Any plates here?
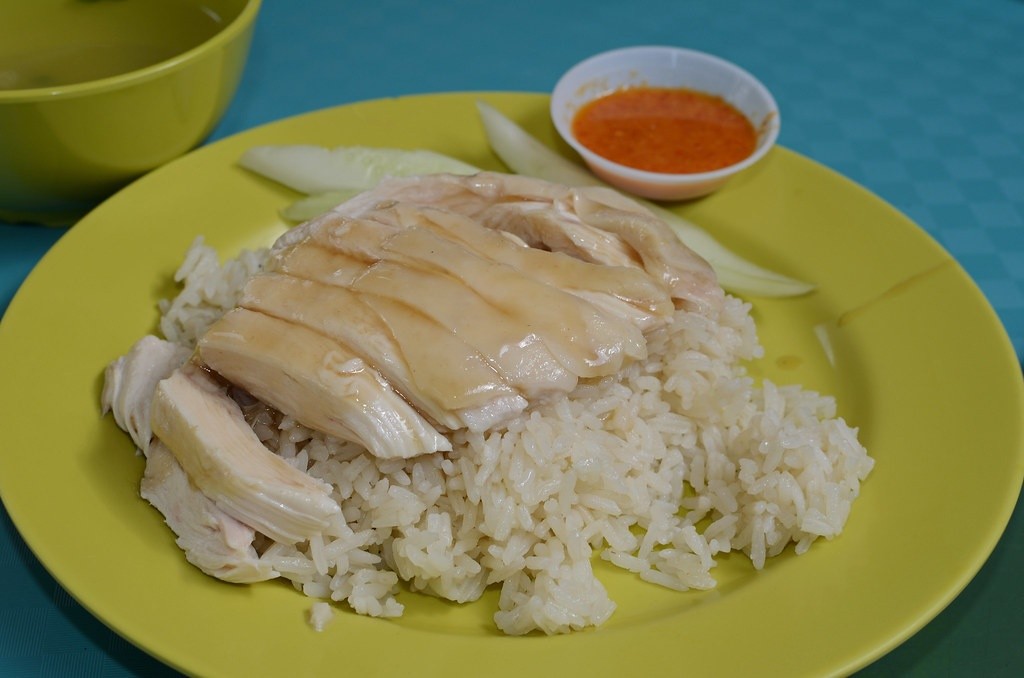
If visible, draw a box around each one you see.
[0,90,1024,678]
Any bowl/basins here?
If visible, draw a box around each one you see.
[0,0,261,227]
[549,45,781,200]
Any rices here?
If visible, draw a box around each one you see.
[155,218,878,637]
